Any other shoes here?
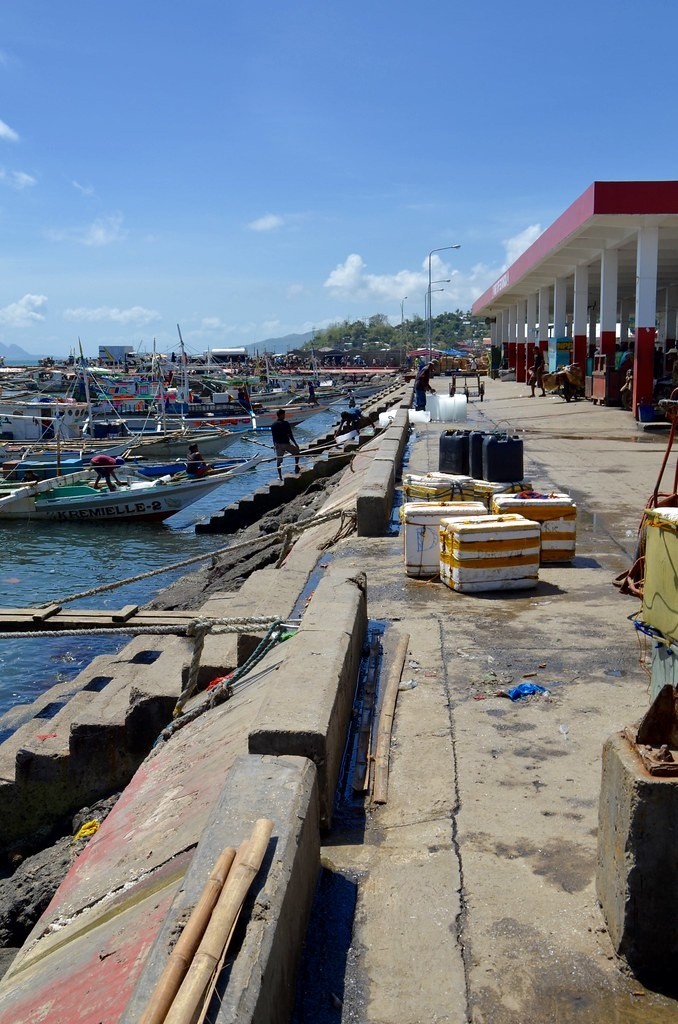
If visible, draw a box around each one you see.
[529,395,535,397]
[539,394,546,397]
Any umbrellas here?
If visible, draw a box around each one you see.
[440,349,467,357]
[318,347,340,355]
[406,349,442,358]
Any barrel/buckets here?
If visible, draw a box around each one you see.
[437,428,524,482]
[637,402,654,422]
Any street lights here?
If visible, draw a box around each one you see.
[427,244,462,380]
[423,288,444,364]
[399,296,409,367]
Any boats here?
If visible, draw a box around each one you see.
[0,321,395,523]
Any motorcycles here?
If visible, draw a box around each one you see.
[554,363,581,402]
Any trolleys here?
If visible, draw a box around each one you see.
[450,372,485,404]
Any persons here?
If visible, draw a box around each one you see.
[20,470,41,482]
[334,408,375,439]
[273,356,377,369]
[526,346,545,397]
[270,409,299,481]
[470,358,477,371]
[307,381,318,407]
[90,455,124,492]
[38,357,266,376]
[185,443,210,479]
[414,359,439,412]
[238,388,246,404]
[613,342,664,412]
[345,389,355,408]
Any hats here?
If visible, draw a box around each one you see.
[116,458,125,465]
[428,359,440,367]
